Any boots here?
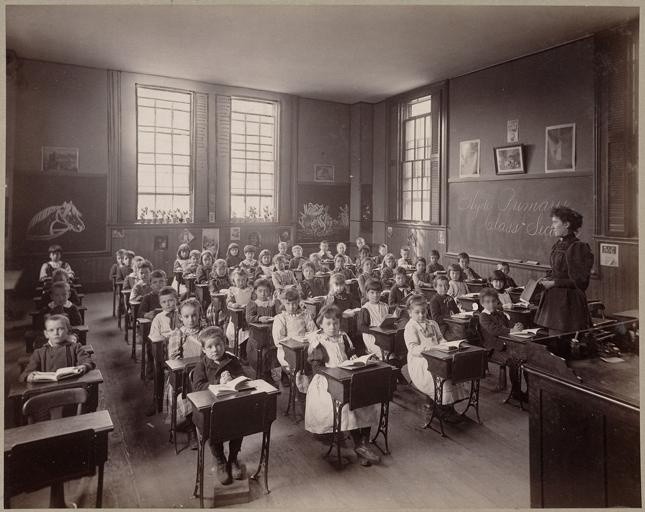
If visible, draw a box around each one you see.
[228,448,244,478]
[216,452,231,485]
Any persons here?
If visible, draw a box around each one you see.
[533,206,595,337]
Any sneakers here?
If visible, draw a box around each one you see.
[512,391,528,402]
[281,371,291,388]
[266,377,281,394]
[189,431,198,450]
[143,402,162,417]
[354,435,381,466]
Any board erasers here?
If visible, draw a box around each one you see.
[527,261,540,265]
[513,259,523,263]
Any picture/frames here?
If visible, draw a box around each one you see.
[492,143,527,176]
[312,163,336,184]
[39,145,80,174]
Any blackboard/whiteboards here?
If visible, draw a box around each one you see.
[10,171,112,256]
[443,168,601,280]
[296,183,373,246]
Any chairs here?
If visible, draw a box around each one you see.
[370,246,639,436]
[4,246,400,510]
